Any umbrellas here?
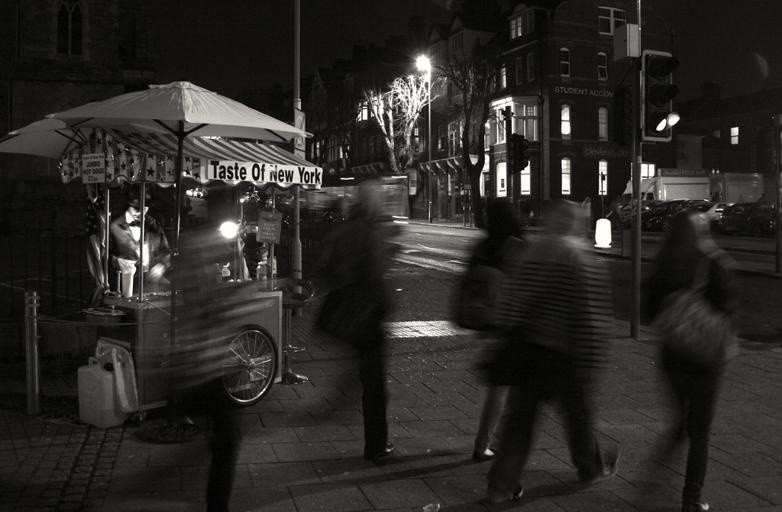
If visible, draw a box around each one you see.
[44,81,313,420]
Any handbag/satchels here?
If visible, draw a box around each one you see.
[655,290,731,373]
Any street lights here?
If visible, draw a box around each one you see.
[414,52,432,221]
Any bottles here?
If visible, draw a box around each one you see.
[221,264,230,284]
[257,261,266,281]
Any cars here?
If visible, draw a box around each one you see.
[594,197,781,232]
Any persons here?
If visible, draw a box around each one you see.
[646,203,735,512]
[312,179,398,465]
[110,193,172,297]
[468,194,620,505]
[176,186,253,511]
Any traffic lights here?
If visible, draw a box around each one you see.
[641,49,683,142]
[508,132,530,173]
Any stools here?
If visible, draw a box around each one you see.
[260,276,315,387]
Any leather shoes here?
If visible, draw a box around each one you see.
[681,502,709,512]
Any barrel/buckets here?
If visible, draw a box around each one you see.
[76,356,130,430]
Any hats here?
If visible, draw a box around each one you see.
[129,194,149,207]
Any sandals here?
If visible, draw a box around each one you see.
[473,448,498,461]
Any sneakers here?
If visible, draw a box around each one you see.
[489,485,524,504]
[364,441,396,460]
[577,446,623,488]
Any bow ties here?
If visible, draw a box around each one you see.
[130,220,141,226]
